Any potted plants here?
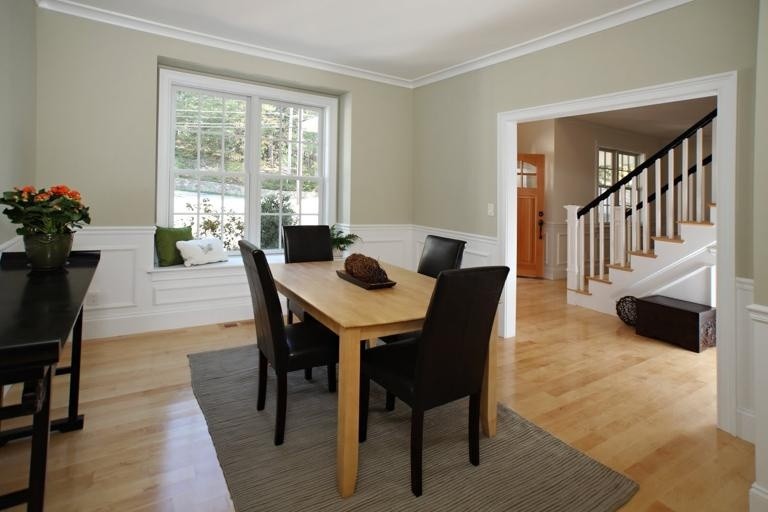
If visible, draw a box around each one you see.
[327,224,357,256]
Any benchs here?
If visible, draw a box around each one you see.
[633,293,715,354]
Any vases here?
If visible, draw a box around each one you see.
[22,230,73,270]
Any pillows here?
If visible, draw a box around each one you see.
[176,236,229,269]
[154,224,193,267]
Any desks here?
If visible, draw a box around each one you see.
[266,257,503,500]
[0,248,103,512]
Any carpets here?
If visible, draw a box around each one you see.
[180,342,639,512]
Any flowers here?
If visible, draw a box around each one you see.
[0,186,92,234]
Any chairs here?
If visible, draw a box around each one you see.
[373,233,468,417]
[233,237,366,444]
[279,224,334,382]
[359,264,510,496]
